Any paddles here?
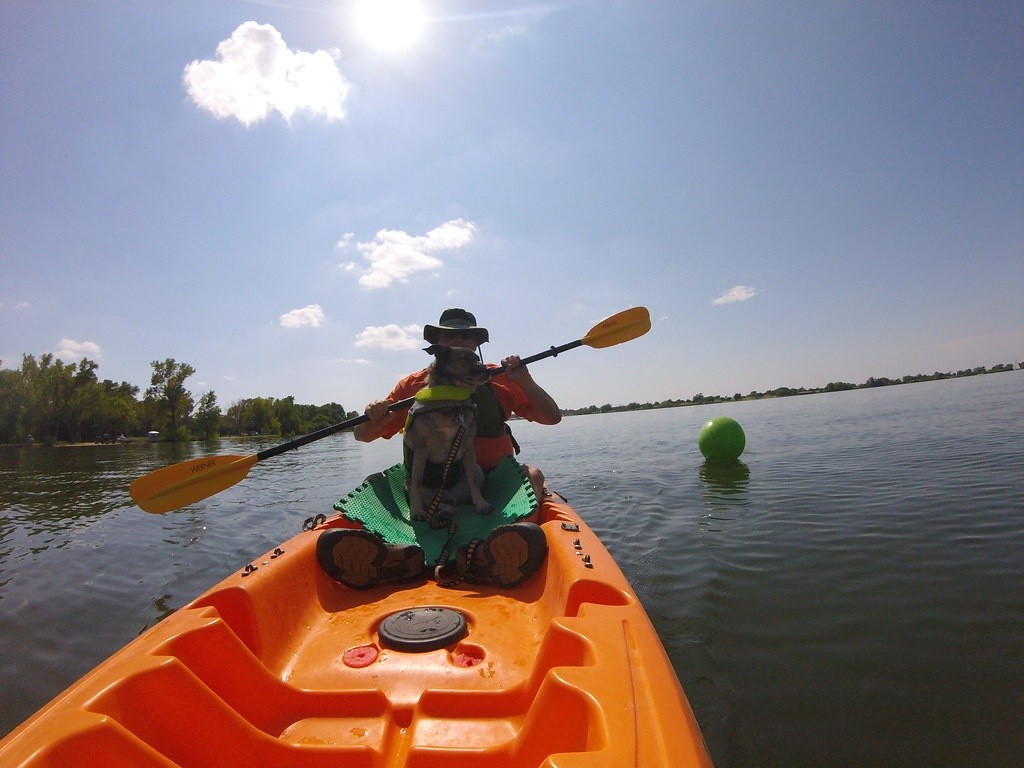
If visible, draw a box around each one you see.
[130,304,652,516]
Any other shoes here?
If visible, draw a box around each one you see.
[449,521,547,589]
[316,528,424,590]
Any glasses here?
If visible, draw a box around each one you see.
[438,331,474,341]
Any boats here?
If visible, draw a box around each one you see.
[0,461,716,767]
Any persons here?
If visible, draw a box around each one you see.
[316,308,562,589]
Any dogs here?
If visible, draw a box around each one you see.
[403,344,495,520]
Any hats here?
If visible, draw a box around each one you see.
[423,309,489,345]
